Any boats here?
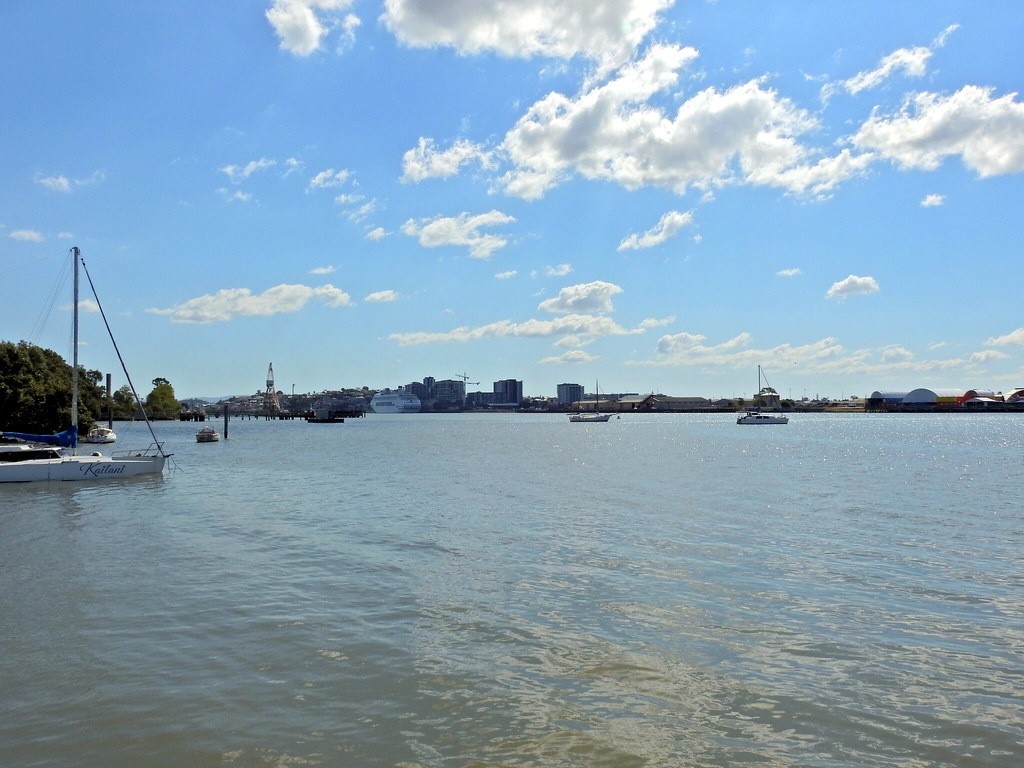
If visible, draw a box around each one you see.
[84,427,117,443]
[195,426,222,442]
[368,386,423,414]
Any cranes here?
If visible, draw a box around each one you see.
[455,372,480,386]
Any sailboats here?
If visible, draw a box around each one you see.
[0,243,176,484]
[569,380,613,422]
[736,365,790,424]
